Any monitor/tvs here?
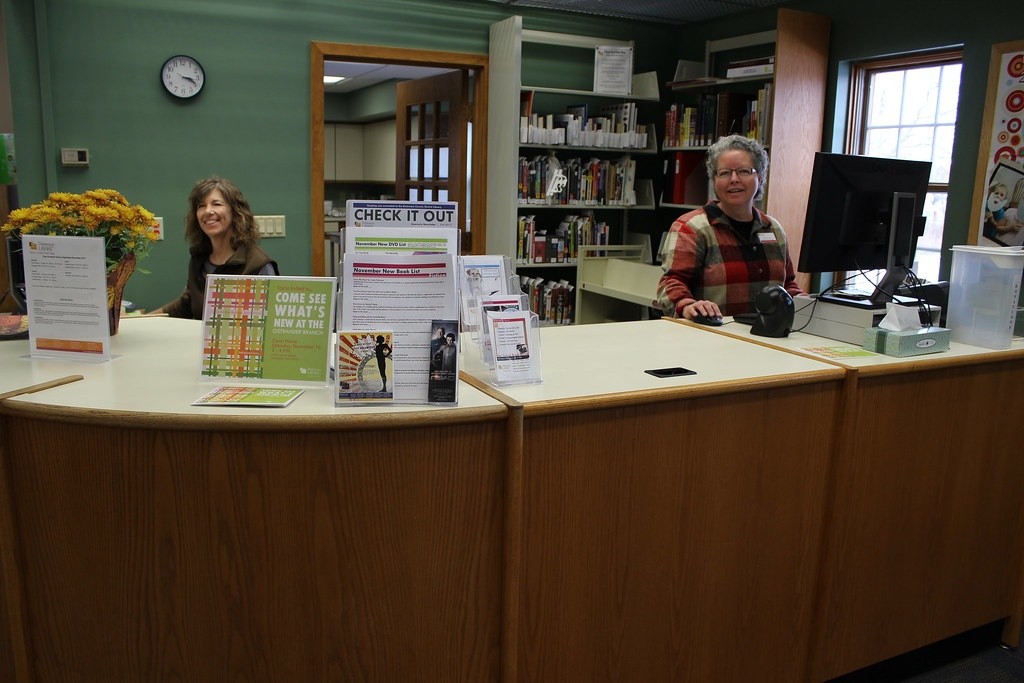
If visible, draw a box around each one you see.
[796,152,933,309]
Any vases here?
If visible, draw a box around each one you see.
[106,251,137,336]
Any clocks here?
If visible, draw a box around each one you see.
[159,55,207,102]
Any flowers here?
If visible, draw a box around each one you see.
[0,187,160,278]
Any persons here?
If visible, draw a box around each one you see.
[120,179,279,320]
[657,135,808,320]
[431,328,457,377]
[983,183,1024,247]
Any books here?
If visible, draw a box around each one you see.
[727,56,775,78]
[518,156,636,206]
[754,172,767,212]
[520,90,647,148]
[518,211,610,263]
[520,277,574,325]
[665,77,724,86]
[708,177,718,201]
[664,83,772,146]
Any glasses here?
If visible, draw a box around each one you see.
[714,168,757,180]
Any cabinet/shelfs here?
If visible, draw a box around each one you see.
[655,8,832,294]
[486,16,661,327]
[323,118,397,184]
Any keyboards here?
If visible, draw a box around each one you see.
[733,312,759,325]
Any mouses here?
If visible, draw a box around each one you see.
[692,309,722,327]
